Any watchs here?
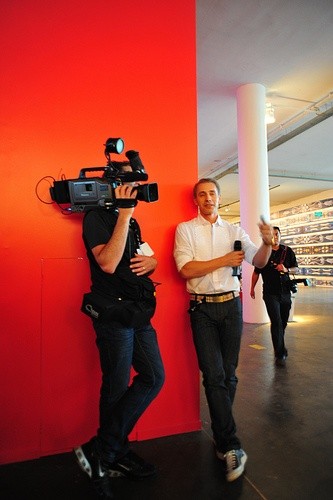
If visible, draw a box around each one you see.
[286,267,291,274]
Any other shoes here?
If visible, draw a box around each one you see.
[277,356,284,366]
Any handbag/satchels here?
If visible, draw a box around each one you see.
[82,292,153,329]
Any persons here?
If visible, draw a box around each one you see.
[249,226,300,367]
[69,159,165,481]
[172,179,274,483]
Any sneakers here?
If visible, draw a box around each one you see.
[74,436,109,480]
[102,451,155,479]
[214,438,225,461]
[224,448,248,481]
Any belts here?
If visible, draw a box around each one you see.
[190,291,239,303]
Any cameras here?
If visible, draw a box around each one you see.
[288,278,309,293]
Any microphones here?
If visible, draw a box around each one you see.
[231,240,242,275]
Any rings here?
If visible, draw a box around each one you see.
[142,266,146,270]
